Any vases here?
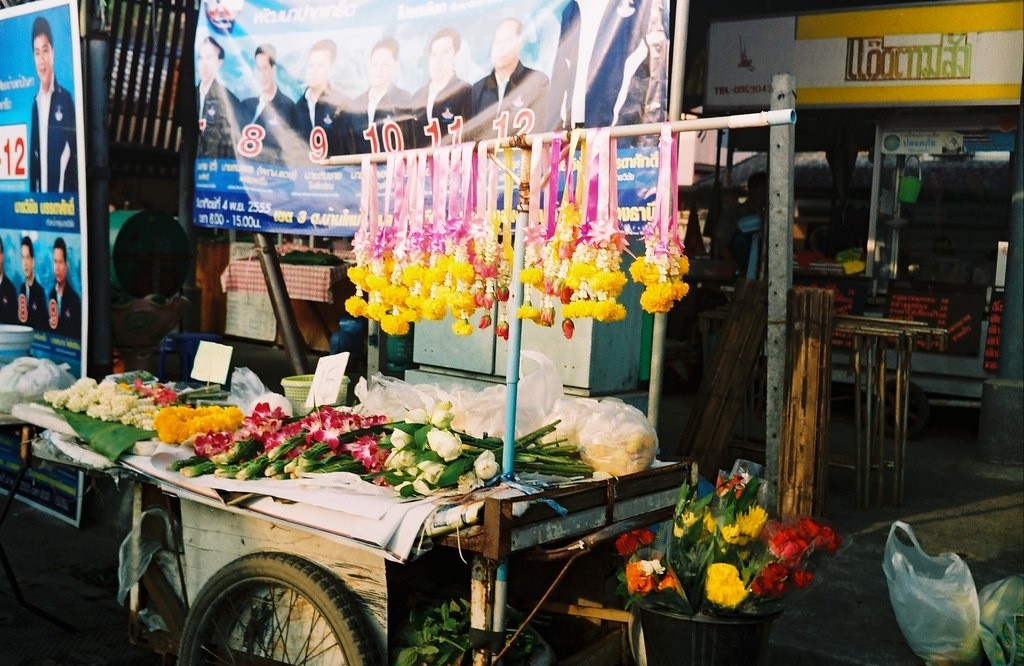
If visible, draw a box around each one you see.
[630,600,784,666]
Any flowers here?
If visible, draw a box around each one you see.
[614,463,841,619]
[345,203,689,340]
[44,375,594,502]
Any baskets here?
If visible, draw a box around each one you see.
[280,374,351,416]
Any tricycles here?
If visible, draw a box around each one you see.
[125,458,700,666]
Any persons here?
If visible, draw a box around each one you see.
[195,1,633,166]
[731,171,768,261]
[0,237,81,341]
[30,17,78,193]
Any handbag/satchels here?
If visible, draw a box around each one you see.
[354,349,659,476]
[882,520,1024,666]
